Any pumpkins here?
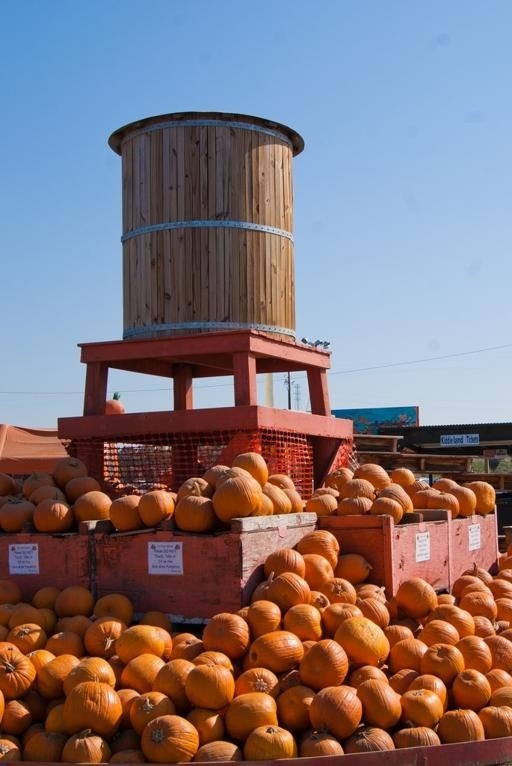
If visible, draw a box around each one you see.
[0,451,512,766]
[104,391,125,414]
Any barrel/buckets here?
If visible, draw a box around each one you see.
[105,111,306,340]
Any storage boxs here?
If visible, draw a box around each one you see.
[317,505,450,606]
[88,510,318,621]
[3,530,90,599]
[450,505,497,594]
